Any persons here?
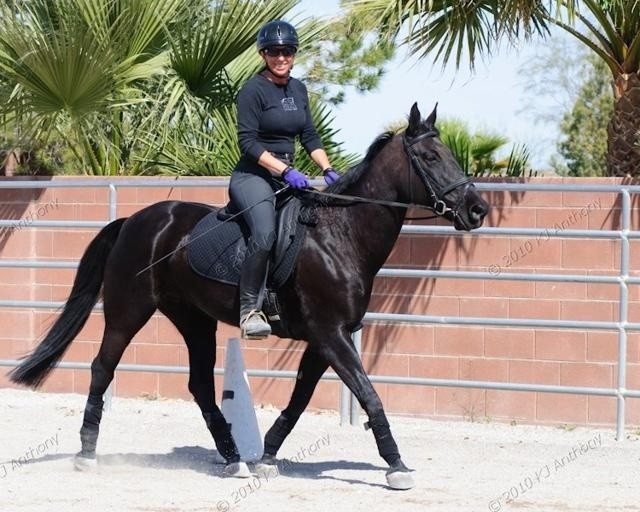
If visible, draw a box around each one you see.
[228,20,339,335]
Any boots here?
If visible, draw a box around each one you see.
[239,245,272,334]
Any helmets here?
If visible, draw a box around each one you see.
[257,21,298,53]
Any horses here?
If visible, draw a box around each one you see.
[6,102,488,491]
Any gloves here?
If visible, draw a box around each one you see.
[282,167,310,188]
[324,171,339,185]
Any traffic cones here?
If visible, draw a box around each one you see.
[215,339,264,465]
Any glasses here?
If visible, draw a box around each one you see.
[265,47,295,56]
